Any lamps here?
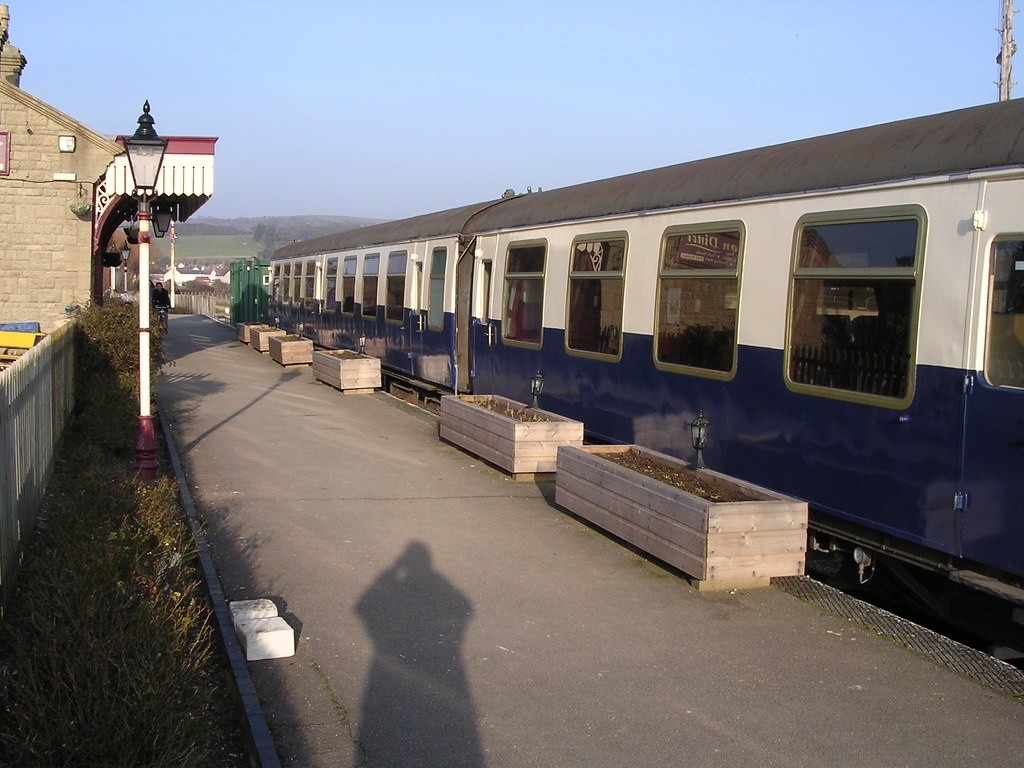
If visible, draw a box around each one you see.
[357,330,368,355]
[297,320,305,339]
[273,313,280,330]
[684,409,713,470]
[155,202,173,232]
[523,370,545,410]
[151,218,164,238]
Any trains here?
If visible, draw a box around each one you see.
[267,95,1023,610]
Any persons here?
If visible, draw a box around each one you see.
[149,279,170,335]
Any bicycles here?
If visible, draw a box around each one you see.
[154,302,170,333]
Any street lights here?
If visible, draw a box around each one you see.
[119,102,165,482]
